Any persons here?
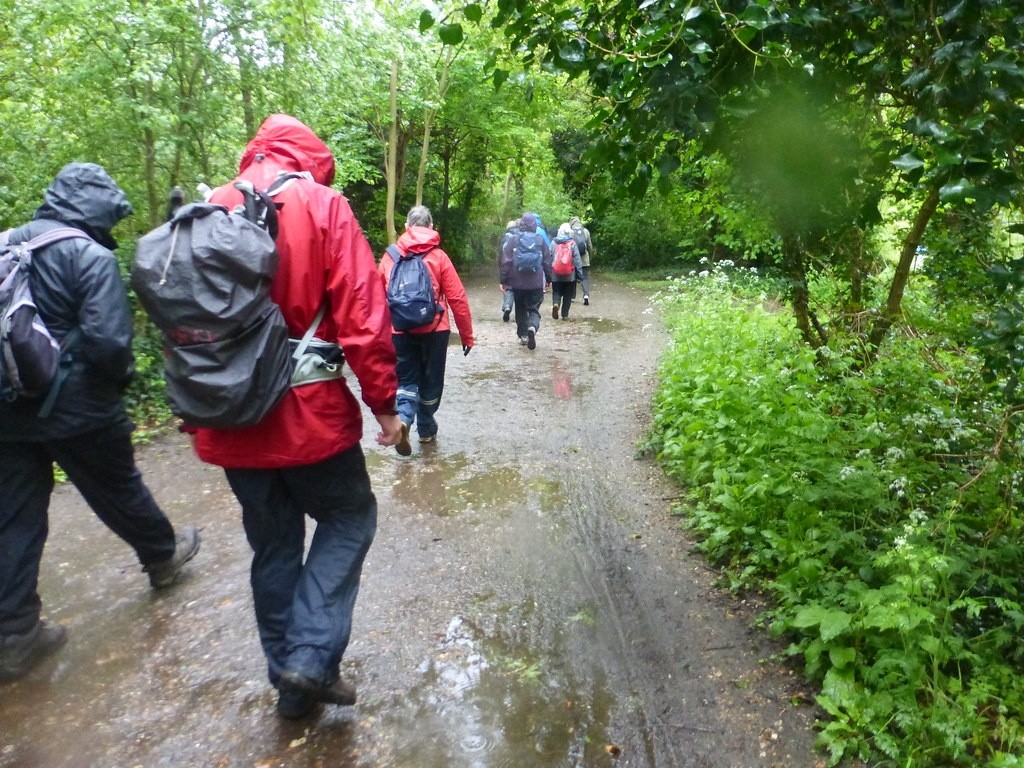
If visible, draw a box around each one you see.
[499,212,593,350]
[379,206,475,456]
[179,113,401,724]
[0,162,202,682]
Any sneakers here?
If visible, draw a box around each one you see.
[1,620,67,682]
[140,523,201,589]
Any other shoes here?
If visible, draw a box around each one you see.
[418,433,437,443]
[395,422,412,456]
[276,696,318,719]
[562,316,568,320]
[527,326,536,351]
[552,303,559,319]
[502,308,510,322]
[583,295,590,306]
[571,299,574,302]
[517,336,528,345]
[281,666,356,704]
[543,289,546,293]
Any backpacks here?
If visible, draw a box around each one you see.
[1,226,97,419]
[573,224,586,255]
[130,171,328,429]
[385,244,444,342]
[515,232,541,274]
[552,239,575,276]
